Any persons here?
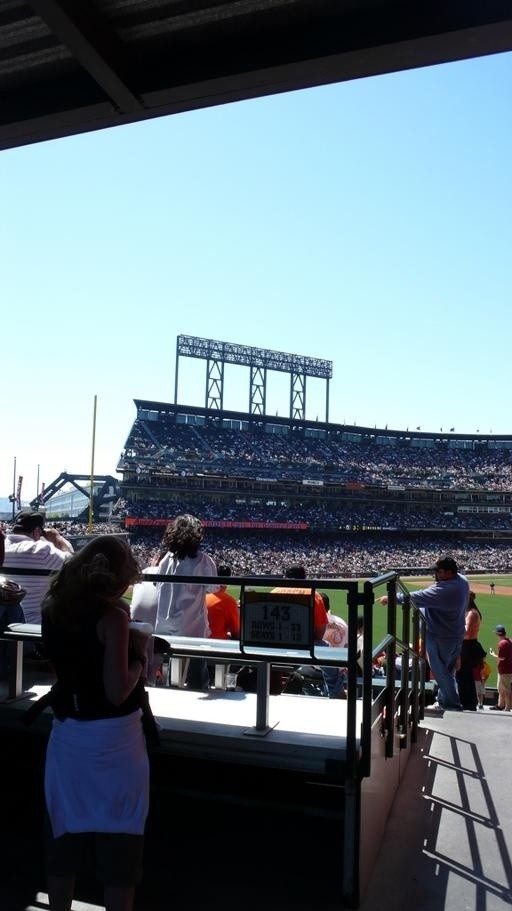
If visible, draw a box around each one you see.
[481,660,493,699]
[1,416,512,579]
[0,513,76,651]
[315,591,438,699]
[269,562,348,700]
[489,579,496,597]
[373,555,472,713]
[34,534,160,911]
[455,589,484,713]
[152,512,219,690]
[487,623,512,711]
[473,657,486,709]
[0,531,40,684]
[203,562,242,642]
[128,548,170,639]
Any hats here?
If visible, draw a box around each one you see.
[15,511,46,530]
[492,625,506,634]
[428,556,457,570]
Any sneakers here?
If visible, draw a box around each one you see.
[478,704,511,711]
[426,702,462,711]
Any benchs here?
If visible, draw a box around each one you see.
[4,622,434,911]
